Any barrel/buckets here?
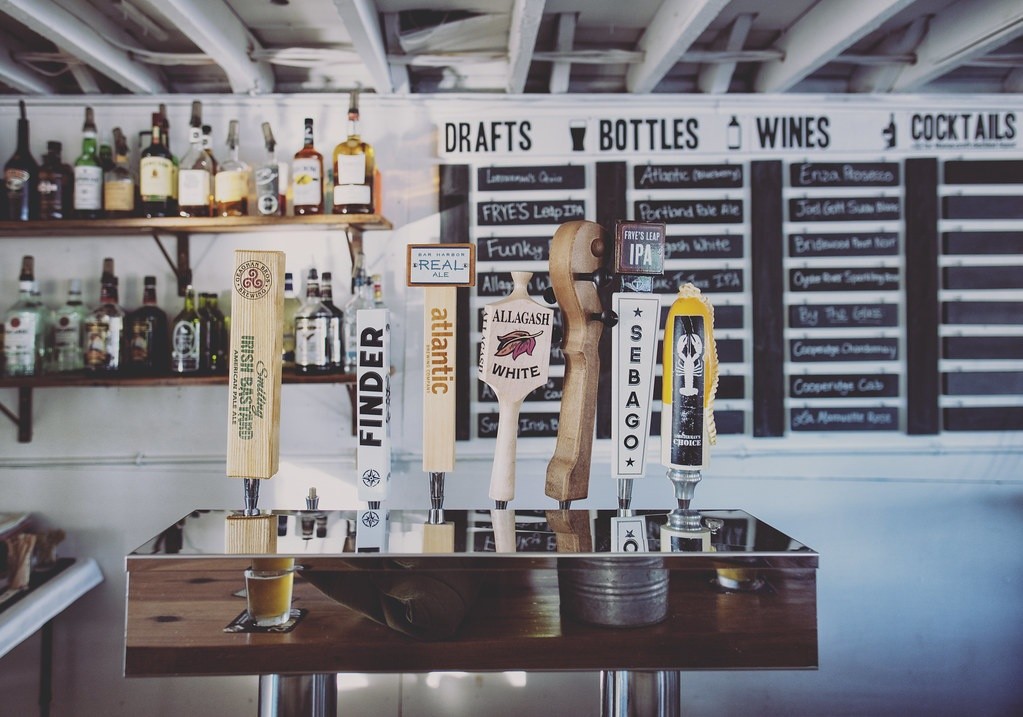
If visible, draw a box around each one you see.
[567,561,669,629]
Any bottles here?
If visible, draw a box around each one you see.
[0,255,225,381]
[281,269,385,374]
[0,100,383,222]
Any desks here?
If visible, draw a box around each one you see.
[0,555,102,717]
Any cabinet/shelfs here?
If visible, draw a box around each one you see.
[0,214,394,445]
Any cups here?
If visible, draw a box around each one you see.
[242,557,294,627]
